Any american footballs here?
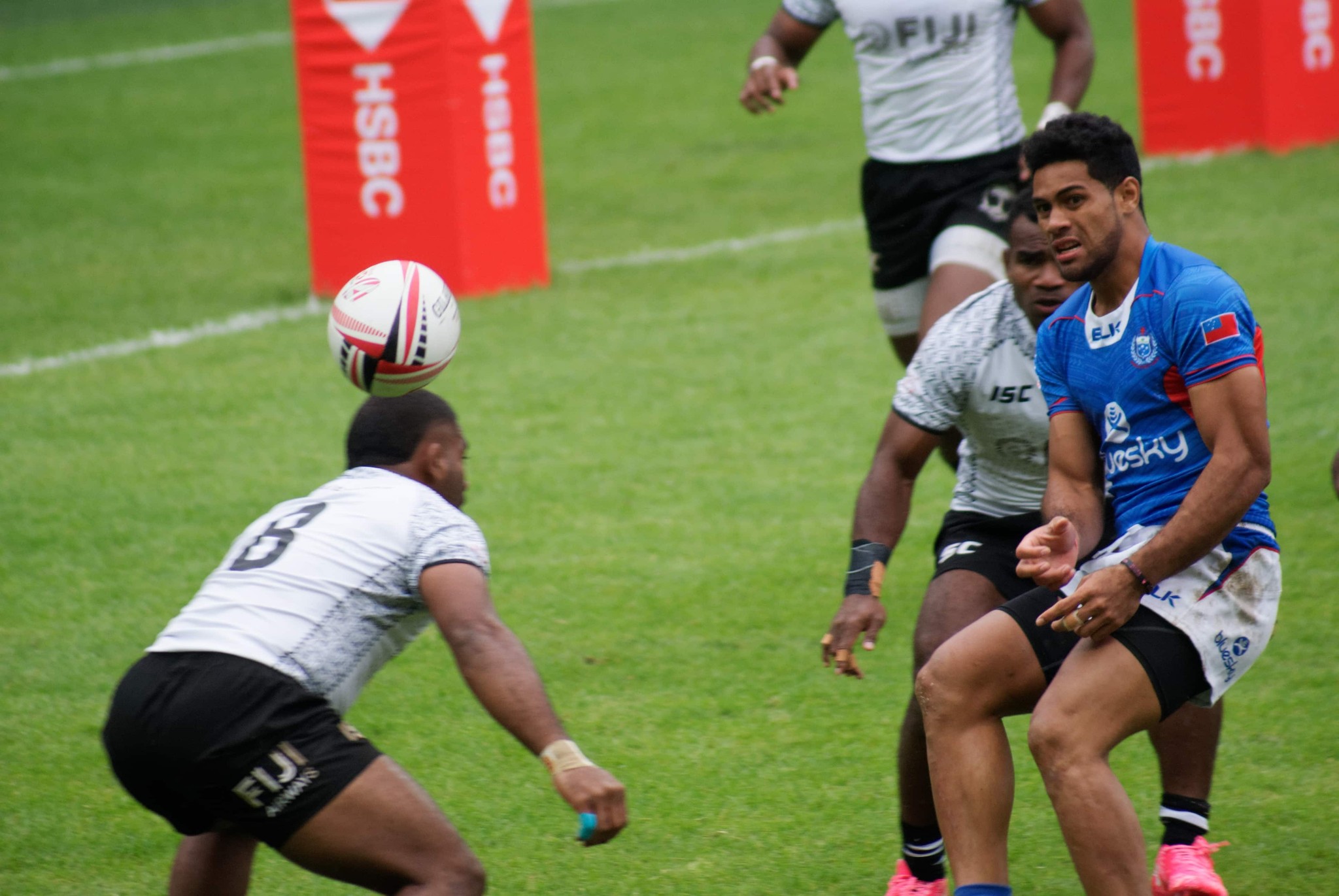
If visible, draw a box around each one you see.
[325,259,464,400]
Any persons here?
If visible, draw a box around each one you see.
[97,386,628,896]
[915,113,1285,896]
[739,0,1097,470]
[823,187,1227,896]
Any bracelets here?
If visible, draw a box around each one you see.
[747,57,782,71]
[1120,557,1153,595]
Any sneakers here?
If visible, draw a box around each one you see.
[1150,830,1231,895]
[886,855,946,895]
